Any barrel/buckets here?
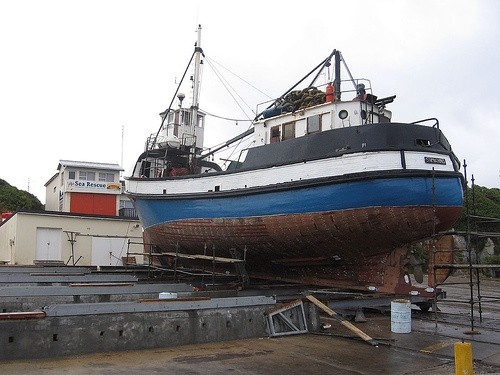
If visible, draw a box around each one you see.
[389,297,413,333]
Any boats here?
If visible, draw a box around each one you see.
[123,22,465,263]
